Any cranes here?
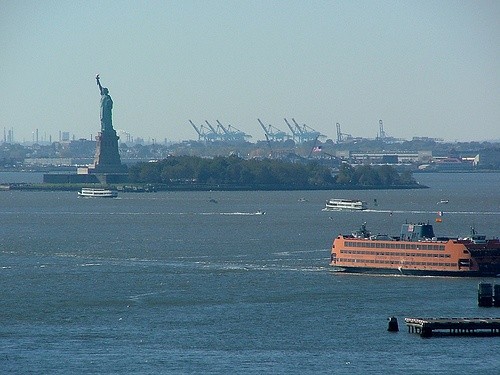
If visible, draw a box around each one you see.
[187,116,386,145]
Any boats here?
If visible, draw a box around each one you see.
[328,220,500,276]
[325,198,367,213]
[77,187,119,199]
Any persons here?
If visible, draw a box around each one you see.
[96,76,113,131]
